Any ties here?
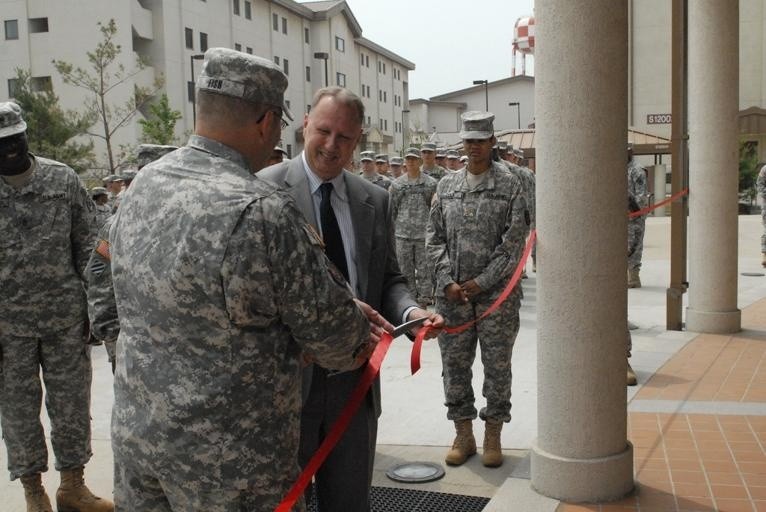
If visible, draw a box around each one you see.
[319,183,351,286]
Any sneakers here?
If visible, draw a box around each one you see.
[626,362,637,386]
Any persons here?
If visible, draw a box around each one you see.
[756,164,766,270]
[107,45,398,510]
[83,145,178,375]
[626,148,647,385]
[1,98,118,511]
[249,83,448,509]
[425,105,532,469]
[268,146,288,167]
[493,139,537,278]
[357,139,467,310]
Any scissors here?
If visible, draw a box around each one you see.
[390,317,429,339]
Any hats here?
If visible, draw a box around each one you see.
[0,102,28,139]
[446,150,459,160]
[435,148,448,158]
[360,150,376,162]
[136,142,180,169]
[458,110,495,141]
[460,155,469,163]
[375,154,389,163]
[91,169,138,196]
[196,46,295,122]
[420,142,436,152]
[389,157,404,166]
[403,147,421,159]
[491,138,525,160]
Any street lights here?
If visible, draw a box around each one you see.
[472,79,489,112]
[508,101,521,129]
[314,51,329,88]
[400,107,411,157]
[190,53,206,134]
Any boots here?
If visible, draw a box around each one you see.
[19,471,54,512]
[445,419,477,465]
[481,416,504,467]
[627,267,642,289]
[56,464,115,512]
